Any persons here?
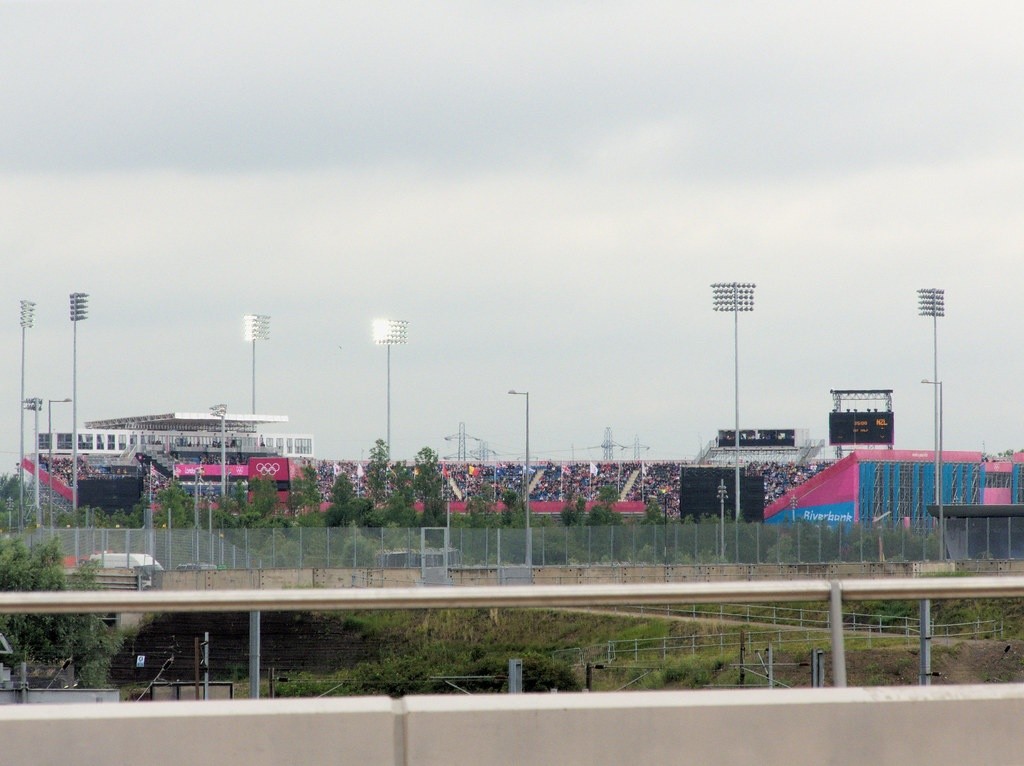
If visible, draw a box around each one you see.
[302,458,539,504]
[261,442,265,446]
[199,440,254,465]
[745,460,834,507]
[155,440,162,445]
[530,462,681,517]
[38,455,172,495]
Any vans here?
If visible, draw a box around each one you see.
[174,563,217,571]
[90,553,164,591]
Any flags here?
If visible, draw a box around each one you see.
[590,462,598,476]
[333,464,342,475]
[443,465,449,478]
[642,463,647,476]
[357,465,364,478]
[620,464,623,472]
[496,468,503,475]
[562,465,571,474]
[469,466,479,476]
[523,466,535,475]
[415,467,421,476]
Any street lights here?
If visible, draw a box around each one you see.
[711,282,756,523]
[922,378,945,561]
[5,498,14,530]
[69,291,89,512]
[244,314,273,415]
[917,288,945,505]
[23,397,43,507]
[19,299,36,506]
[372,318,409,460]
[716,485,729,560]
[209,404,229,497]
[47,399,73,528]
[508,390,529,529]
